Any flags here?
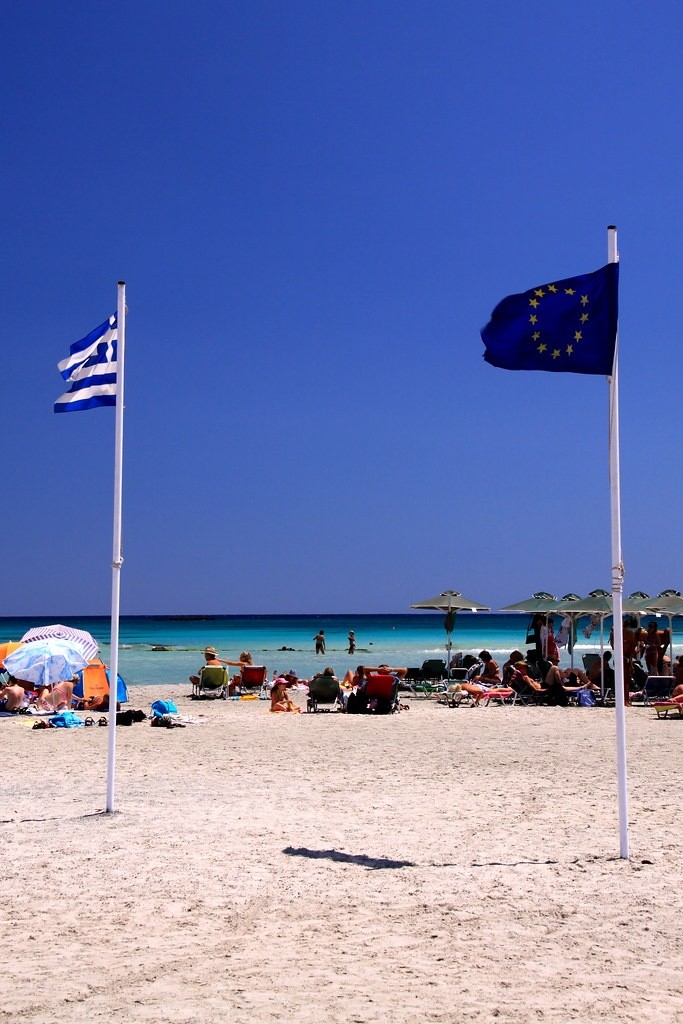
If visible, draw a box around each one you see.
[52,311,118,414]
[480,262,620,376]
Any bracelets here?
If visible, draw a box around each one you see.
[392,668,393,672]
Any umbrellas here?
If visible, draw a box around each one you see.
[3,638,100,711]
[21,623,101,661]
[409,589,492,674]
[498,589,683,696]
[0,639,26,669]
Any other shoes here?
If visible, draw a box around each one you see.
[32,721,46,729]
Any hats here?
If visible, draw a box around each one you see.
[276,678,289,685]
[200,646,219,655]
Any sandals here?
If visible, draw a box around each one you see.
[85,717,93,725]
[99,717,107,726]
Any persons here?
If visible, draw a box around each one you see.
[313,630,325,654]
[347,630,356,654]
[272,664,410,714]
[0,672,121,712]
[216,650,255,685]
[447,613,683,715]
[269,678,301,713]
[189,646,224,686]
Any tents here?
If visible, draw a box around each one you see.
[71,657,129,705]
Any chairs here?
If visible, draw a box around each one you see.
[307,675,344,713]
[358,651,677,714]
[232,665,267,698]
[192,666,229,701]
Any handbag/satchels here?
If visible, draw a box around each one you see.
[577,689,597,707]
